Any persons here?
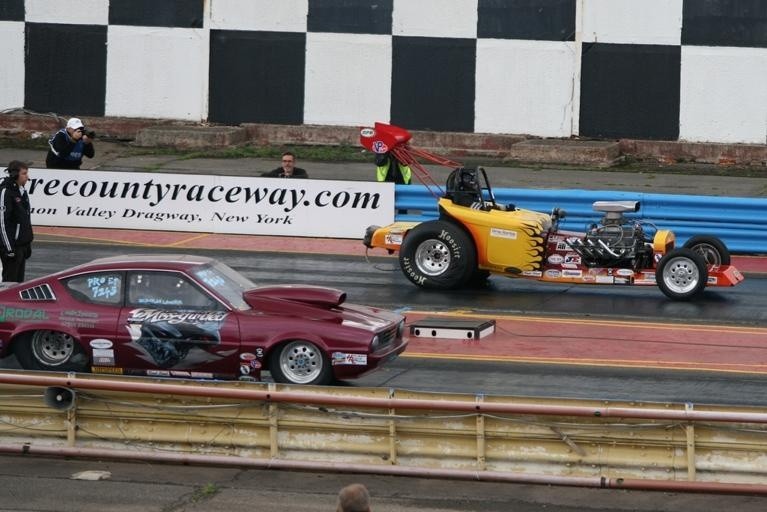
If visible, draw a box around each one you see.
[259,151,308,178]
[44,116,94,169]
[448,162,516,214]
[0,160,33,282]
[372,140,413,214]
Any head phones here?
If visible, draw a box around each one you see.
[10,161,22,180]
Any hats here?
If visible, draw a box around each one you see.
[66,117,86,130]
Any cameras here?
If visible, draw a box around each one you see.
[77,130,95,138]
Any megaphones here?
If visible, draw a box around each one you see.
[45,385,76,411]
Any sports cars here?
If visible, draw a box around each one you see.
[0,252,413,387]
[358,121,747,299]
